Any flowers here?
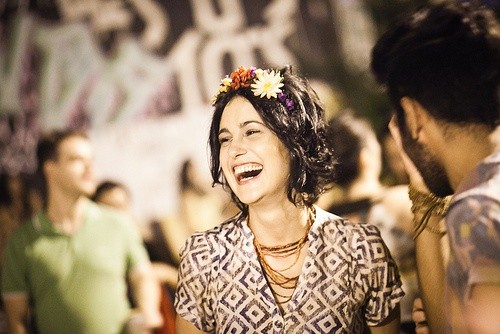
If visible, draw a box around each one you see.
[210,64,285,105]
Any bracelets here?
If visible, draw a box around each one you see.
[408,184,448,241]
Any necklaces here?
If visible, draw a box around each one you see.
[247,206,315,304]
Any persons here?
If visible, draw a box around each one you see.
[179,158,231,243]
[371,0,500,334]
[0,128,163,334]
[173,65,406,334]
[323,114,421,334]
[1,172,136,334]
